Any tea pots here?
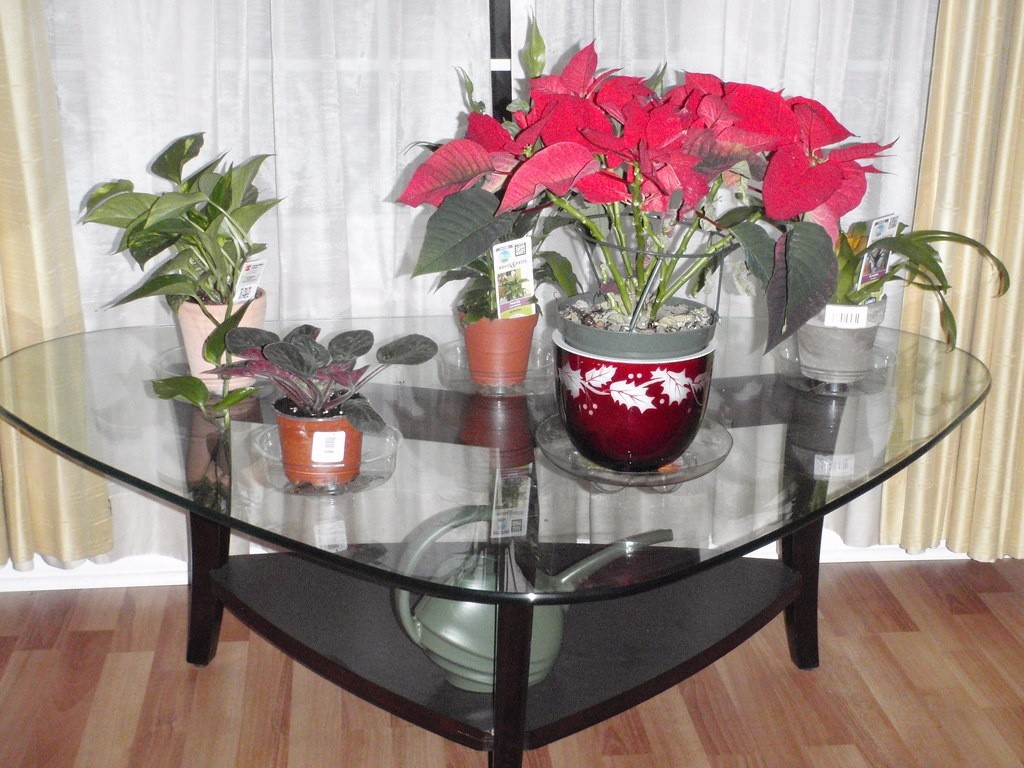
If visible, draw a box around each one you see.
[391,508,676,692]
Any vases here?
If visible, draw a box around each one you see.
[458,391,536,470]
[546,290,721,471]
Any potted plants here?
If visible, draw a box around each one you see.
[402,8,557,386]
[728,201,1010,390]
[224,327,442,492]
[83,132,282,405]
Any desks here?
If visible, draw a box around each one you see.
[1,309,989,767]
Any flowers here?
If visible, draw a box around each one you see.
[392,25,898,357]
